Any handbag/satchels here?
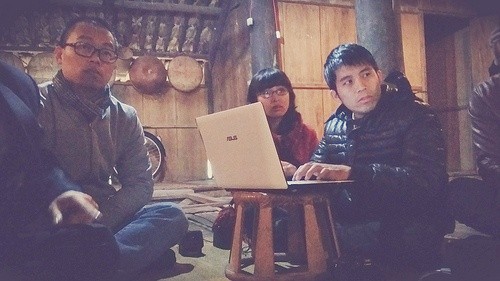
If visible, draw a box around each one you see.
[212,205,237,250]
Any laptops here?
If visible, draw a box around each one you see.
[195,101,354,192]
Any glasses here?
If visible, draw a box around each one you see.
[62,41,120,65]
[257,88,288,98]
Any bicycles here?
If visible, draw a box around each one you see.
[113,129,167,180]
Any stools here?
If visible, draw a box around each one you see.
[225,190,341,281]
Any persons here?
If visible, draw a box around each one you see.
[212,66,319,250]
[449,27,500,238]
[36,16,189,281]
[0,60,119,281]
[281,44,455,281]
